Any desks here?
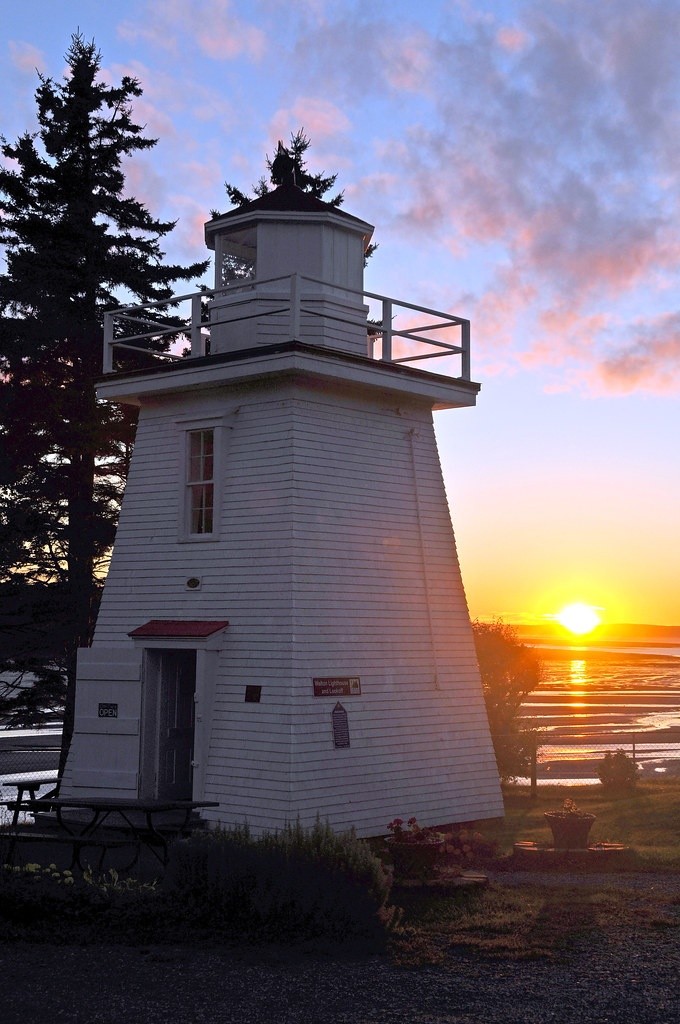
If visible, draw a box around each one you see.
[2,779,62,825]
[0,799,221,874]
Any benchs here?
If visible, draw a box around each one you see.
[0,832,144,879]
[0,800,45,808]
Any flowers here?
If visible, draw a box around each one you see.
[437,831,484,870]
[1,857,159,912]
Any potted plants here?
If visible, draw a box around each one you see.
[544,799,597,847]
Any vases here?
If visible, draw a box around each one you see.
[384,817,444,878]
